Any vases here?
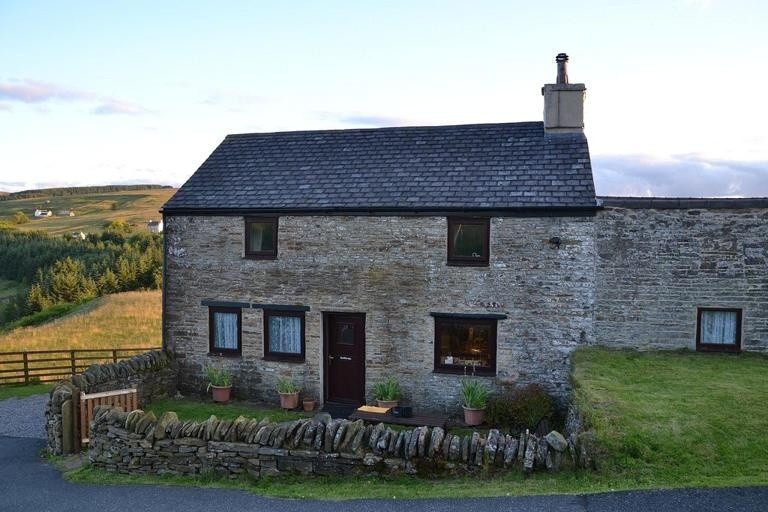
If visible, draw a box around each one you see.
[302,397,317,412]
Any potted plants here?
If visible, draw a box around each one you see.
[460,378,497,427]
[371,377,403,414]
[202,364,235,403]
[273,375,303,410]
